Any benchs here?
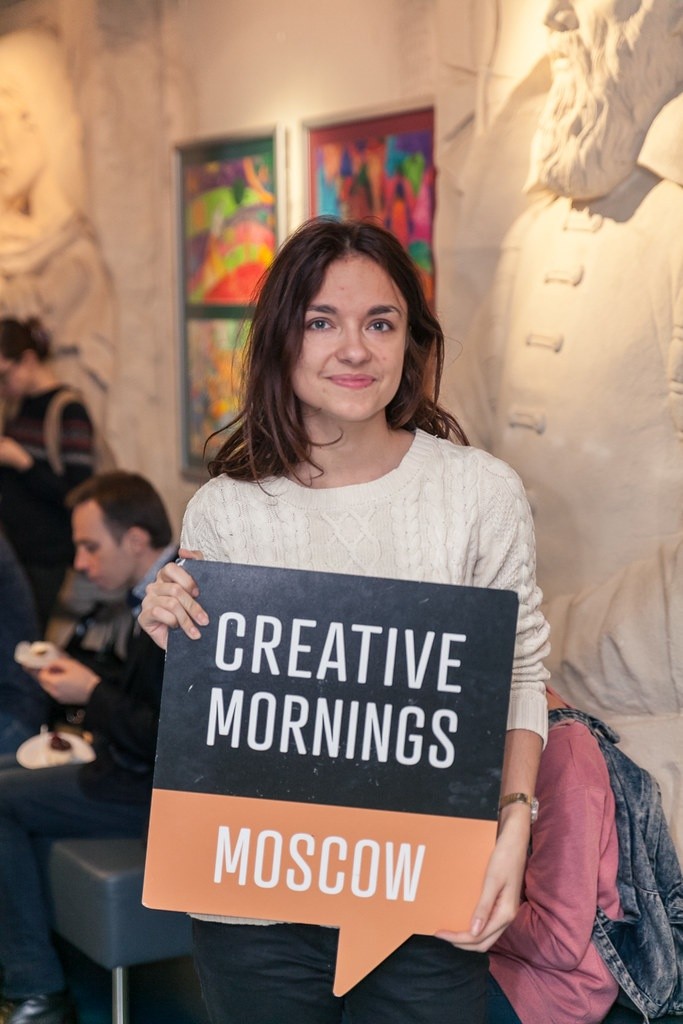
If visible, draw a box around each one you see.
[40,838,192,1024]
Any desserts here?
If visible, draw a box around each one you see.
[43,736,73,766]
[13,639,63,669]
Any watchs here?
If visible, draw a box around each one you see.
[498,793,539,825]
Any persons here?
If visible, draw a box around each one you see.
[0,317,181,1024]
[139,214,683,1024]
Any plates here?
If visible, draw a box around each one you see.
[16,733,95,769]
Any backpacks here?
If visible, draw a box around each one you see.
[46,390,116,485]
[548,707,683,1024]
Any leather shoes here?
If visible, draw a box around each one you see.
[0,986,79,1024]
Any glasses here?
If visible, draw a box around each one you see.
[0,359,19,383]
